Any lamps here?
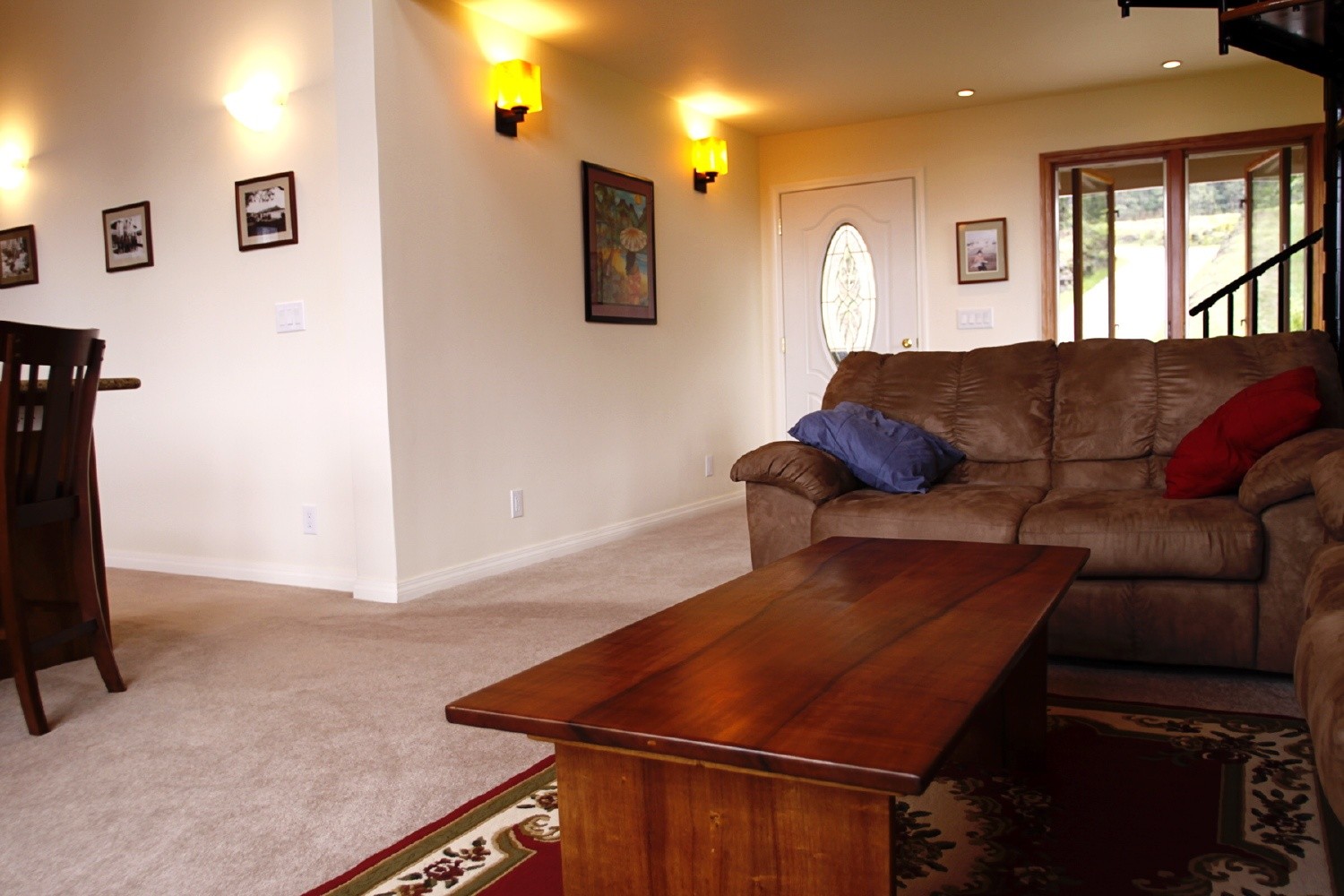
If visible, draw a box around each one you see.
[488,60,542,139]
[692,138,729,195]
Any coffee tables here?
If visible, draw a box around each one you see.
[445,537,1092,895]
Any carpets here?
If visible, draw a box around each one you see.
[298,694,1344,895]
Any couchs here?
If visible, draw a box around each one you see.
[1293,449,1344,825]
[730,330,1344,675]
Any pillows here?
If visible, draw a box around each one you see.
[787,401,966,496]
[1162,367,1320,500]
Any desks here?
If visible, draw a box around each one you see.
[0,379,140,681]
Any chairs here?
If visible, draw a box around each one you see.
[0,319,127,737]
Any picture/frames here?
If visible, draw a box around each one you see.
[956,218,1009,285]
[102,200,154,274]
[234,170,298,253]
[0,224,40,290]
[581,160,658,325]
